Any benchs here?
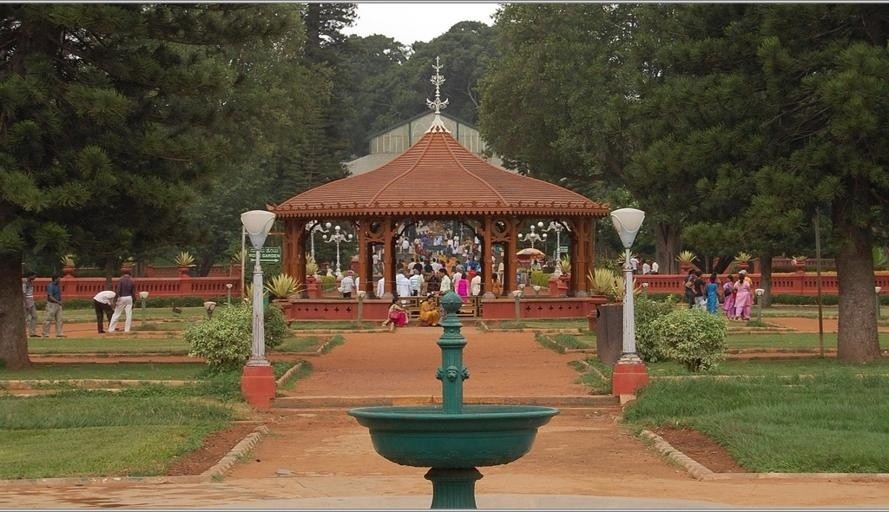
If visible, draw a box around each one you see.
[398,295,511,317]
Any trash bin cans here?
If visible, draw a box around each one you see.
[595,302,623,366]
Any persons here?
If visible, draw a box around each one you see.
[339,230,557,329]
[108,267,136,332]
[42,274,67,337]
[23,271,41,337]
[682,270,754,320]
[631,251,659,274]
[92,290,119,332]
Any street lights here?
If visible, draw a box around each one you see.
[610,206,649,397]
[239,208,277,410]
[322,226,353,275]
[517,225,548,270]
[305,218,332,261]
[538,220,571,277]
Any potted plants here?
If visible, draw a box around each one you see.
[583,264,618,306]
[173,249,197,274]
[262,273,310,309]
[734,250,752,269]
[674,250,695,271]
[59,255,76,273]
[304,262,319,282]
